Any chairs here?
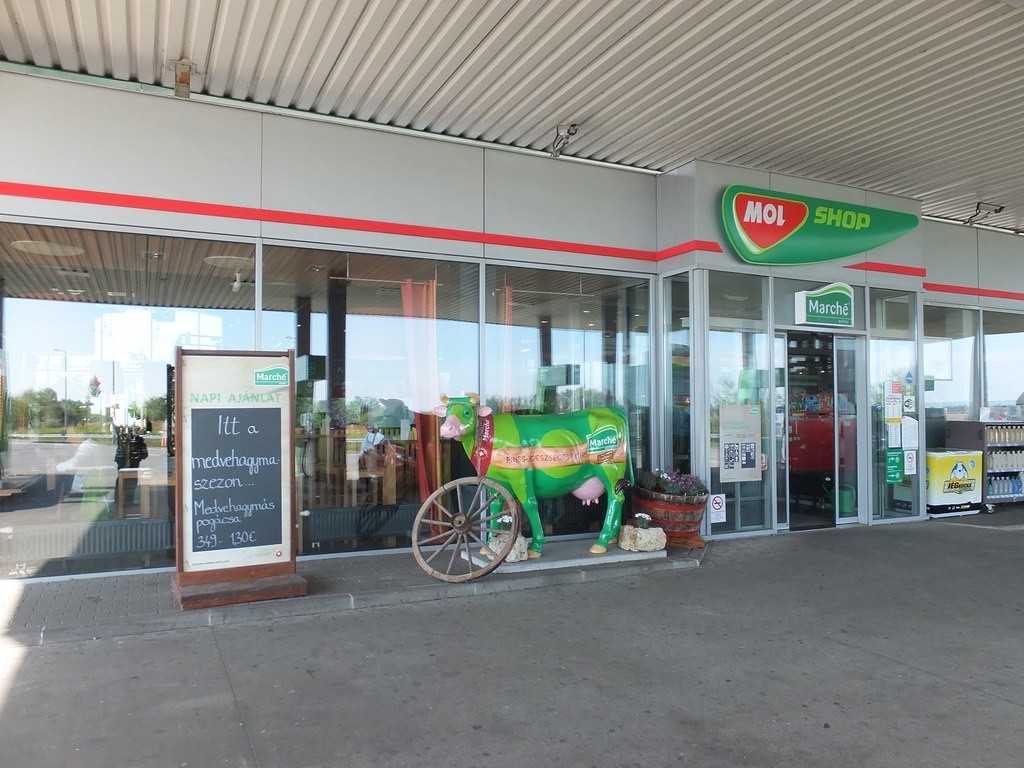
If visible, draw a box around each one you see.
[48,467,114,571]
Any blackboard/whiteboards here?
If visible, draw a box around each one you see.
[191,407,282,553]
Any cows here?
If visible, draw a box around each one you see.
[432,392,635,559]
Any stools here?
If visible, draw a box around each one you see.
[119,468,150,519]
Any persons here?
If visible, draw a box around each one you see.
[114,404,149,508]
[360,418,387,499]
[56,421,106,518]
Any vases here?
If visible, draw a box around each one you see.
[632,485,708,537]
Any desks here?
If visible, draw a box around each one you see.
[295,433,374,521]
[784,419,857,512]
[356,433,418,547]
[138,476,176,519]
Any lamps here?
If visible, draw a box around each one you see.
[109,397,149,468]
[232,273,243,293]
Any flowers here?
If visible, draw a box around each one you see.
[638,466,707,497]
